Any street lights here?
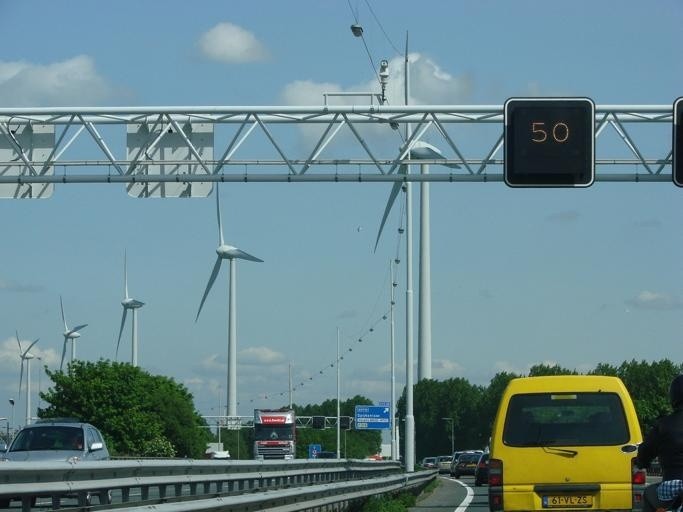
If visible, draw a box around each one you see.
[9,398,15,439]
[441,418,455,455]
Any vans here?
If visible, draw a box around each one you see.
[488,375,647,512]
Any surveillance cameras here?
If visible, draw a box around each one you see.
[381,60,388,71]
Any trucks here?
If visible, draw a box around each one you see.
[253,408,296,461]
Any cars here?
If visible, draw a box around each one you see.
[0,418,113,507]
[422,445,492,486]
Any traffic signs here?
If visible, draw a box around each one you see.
[503,97,595,188]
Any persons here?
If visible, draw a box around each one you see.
[636,373,683,512]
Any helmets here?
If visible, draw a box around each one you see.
[670,374,682,401]
